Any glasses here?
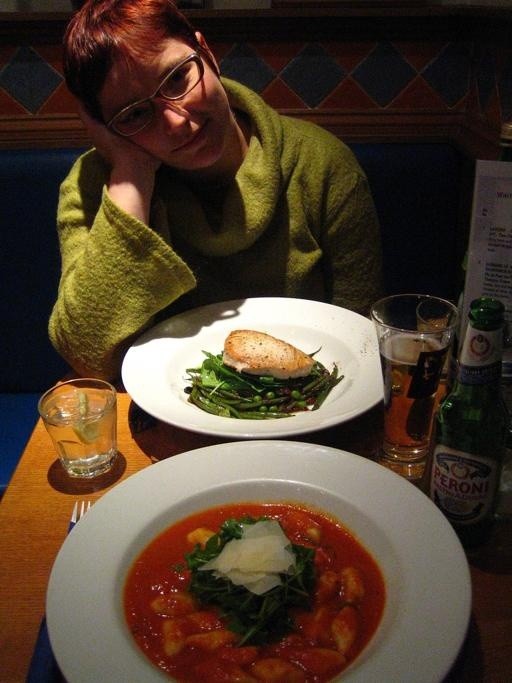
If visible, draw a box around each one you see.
[105,44,204,136]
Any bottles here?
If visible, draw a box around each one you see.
[426,297,510,546]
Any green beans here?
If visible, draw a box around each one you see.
[254,369,331,413]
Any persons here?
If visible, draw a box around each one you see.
[45,0,387,386]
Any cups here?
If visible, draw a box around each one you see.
[38,378,118,479]
[369,291,463,479]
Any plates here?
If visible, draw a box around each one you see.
[44,438,474,682]
[119,294,387,438]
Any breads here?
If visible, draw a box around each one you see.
[221,329,315,378]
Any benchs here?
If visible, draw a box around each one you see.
[1,132,476,494]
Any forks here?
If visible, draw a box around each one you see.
[65,500,89,531]
